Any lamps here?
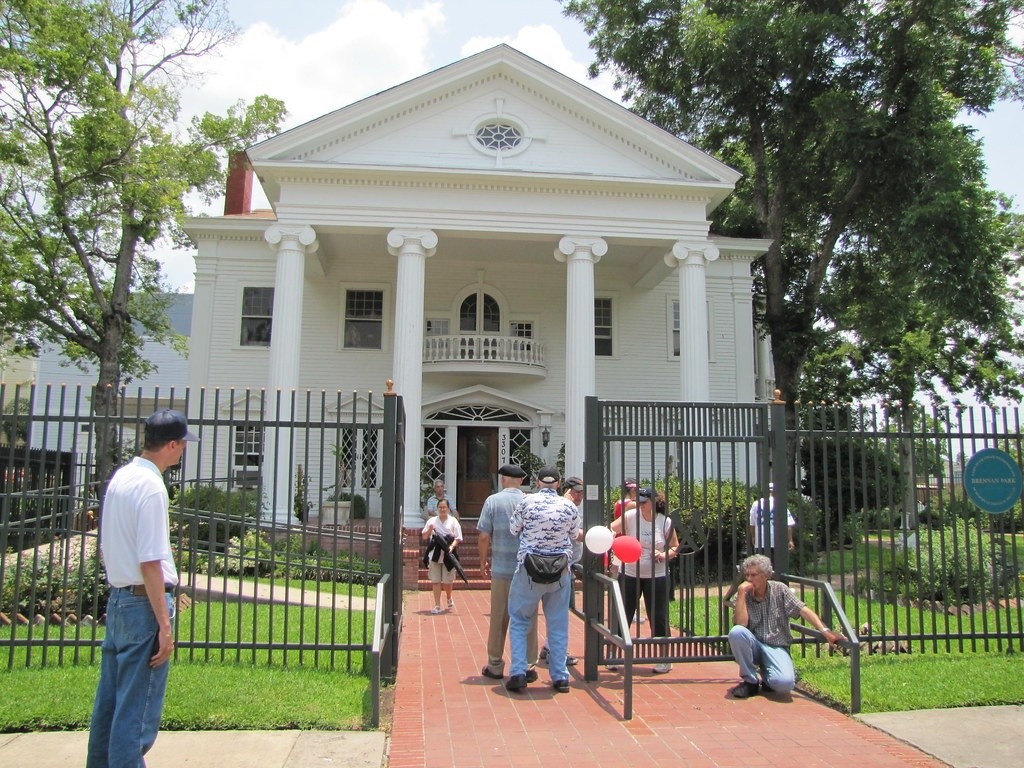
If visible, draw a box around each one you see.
[542,425,550,447]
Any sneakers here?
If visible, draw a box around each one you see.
[604,654,619,670]
[653,662,672,673]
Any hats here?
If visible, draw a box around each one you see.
[498,464,524,477]
[769,482,773,491]
[538,466,560,483]
[143,409,201,441]
[636,487,659,503]
[562,477,584,491]
[625,481,641,488]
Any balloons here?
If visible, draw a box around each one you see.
[613,536,642,563]
[584,525,613,554]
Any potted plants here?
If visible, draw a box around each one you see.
[321,444,351,525]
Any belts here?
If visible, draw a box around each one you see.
[126,584,171,593]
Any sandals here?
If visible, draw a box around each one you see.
[447,597,453,608]
[431,606,441,614]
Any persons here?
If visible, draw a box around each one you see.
[422,499,463,614]
[86,408,200,768]
[427,479,460,521]
[604,487,680,673]
[504,468,579,693]
[476,465,538,682]
[750,482,796,562]
[728,554,847,698]
[614,477,640,537]
[539,477,583,665]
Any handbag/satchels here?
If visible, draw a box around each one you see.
[524,552,568,584]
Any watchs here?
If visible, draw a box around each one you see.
[819,628,830,633]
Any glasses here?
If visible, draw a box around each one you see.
[639,492,652,497]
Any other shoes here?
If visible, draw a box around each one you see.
[482,666,503,678]
[506,674,527,690]
[552,679,570,694]
[525,668,539,683]
[540,647,551,659]
[732,682,759,698]
[762,681,775,692]
[546,650,578,665]
